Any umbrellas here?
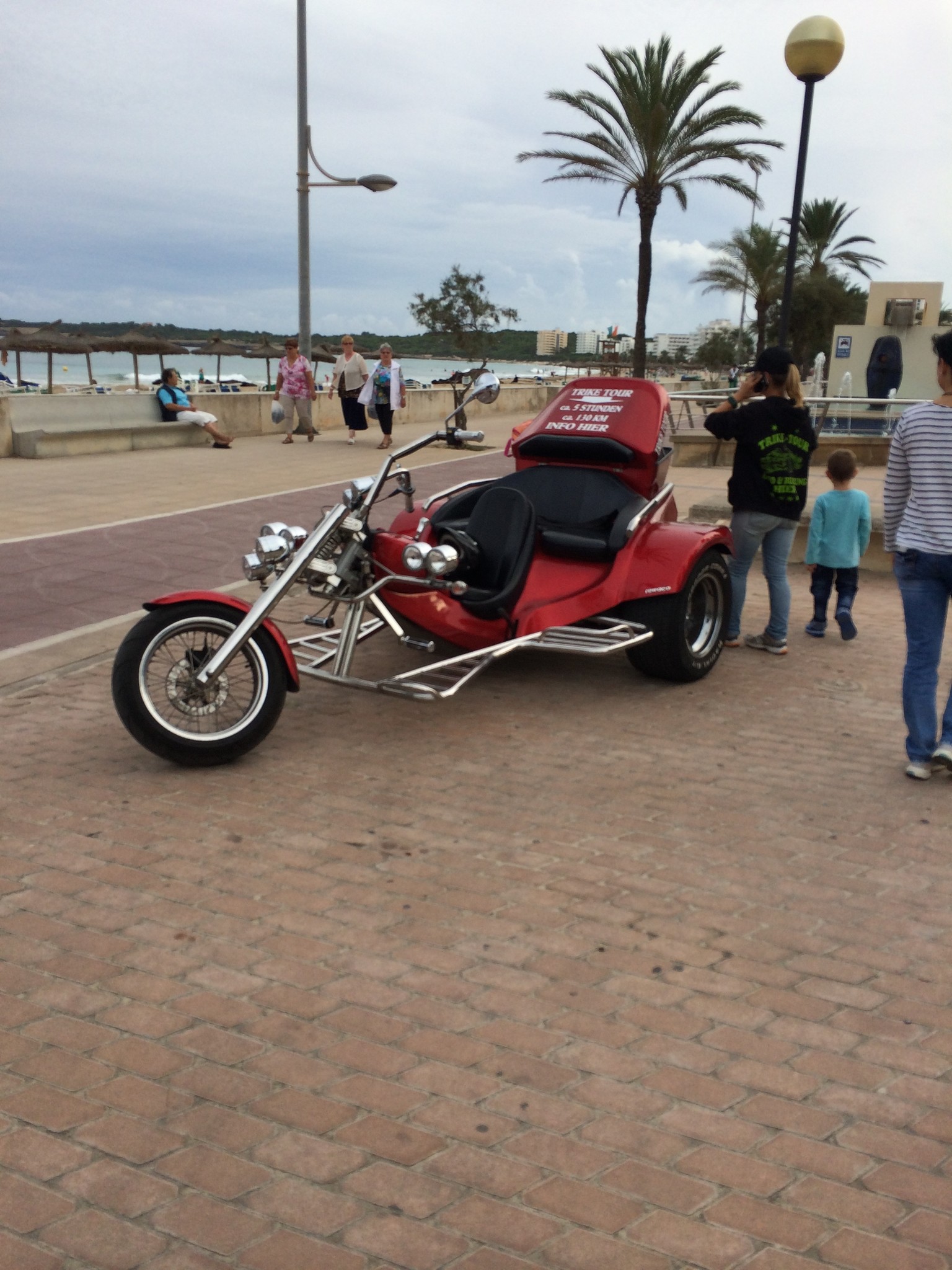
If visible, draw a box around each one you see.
[190,336,246,382]
[241,337,287,392]
[311,344,336,380]
[364,348,404,360]
[0,324,190,395]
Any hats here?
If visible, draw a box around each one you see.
[745,346,794,374]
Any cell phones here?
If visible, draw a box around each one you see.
[753,378,767,393]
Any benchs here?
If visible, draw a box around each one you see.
[433,435,649,554]
[7,396,210,459]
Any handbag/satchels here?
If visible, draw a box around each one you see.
[271,399,284,425]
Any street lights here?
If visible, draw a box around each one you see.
[295,126,400,372]
[776,15,844,354]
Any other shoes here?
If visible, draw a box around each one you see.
[213,442,230,449]
[283,438,293,443]
[347,439,355,445]
[307,432,313,442]
[805,625,824,637]
[377,439,392,449]
[835,607,858,641]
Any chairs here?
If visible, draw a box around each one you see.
[450,486,538,620]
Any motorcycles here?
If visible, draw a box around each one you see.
[109,370,740,768]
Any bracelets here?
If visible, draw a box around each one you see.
[330,387,334,390]
[727,396,738,408]
[274,389,279,392]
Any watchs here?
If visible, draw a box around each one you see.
[401,395,406,399]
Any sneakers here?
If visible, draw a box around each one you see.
[906,760,947,779]
[931,740,952,772]
[743,632,787,654]
[723,631,739,647]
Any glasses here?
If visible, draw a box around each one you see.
[344,342,352,345]
[382,352,390,354]
[284,347,294,350]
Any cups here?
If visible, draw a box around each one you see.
[190,380,199,392]
[322,383,330,391]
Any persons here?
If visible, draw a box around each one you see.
[272,338,317,444]
[328,335,369,445]
[155,368,235,445]
[704,346,819,654]
[325,374,329,383]
[357,342,406,449]
[884,328,952,781]
[803,449,872,642]
